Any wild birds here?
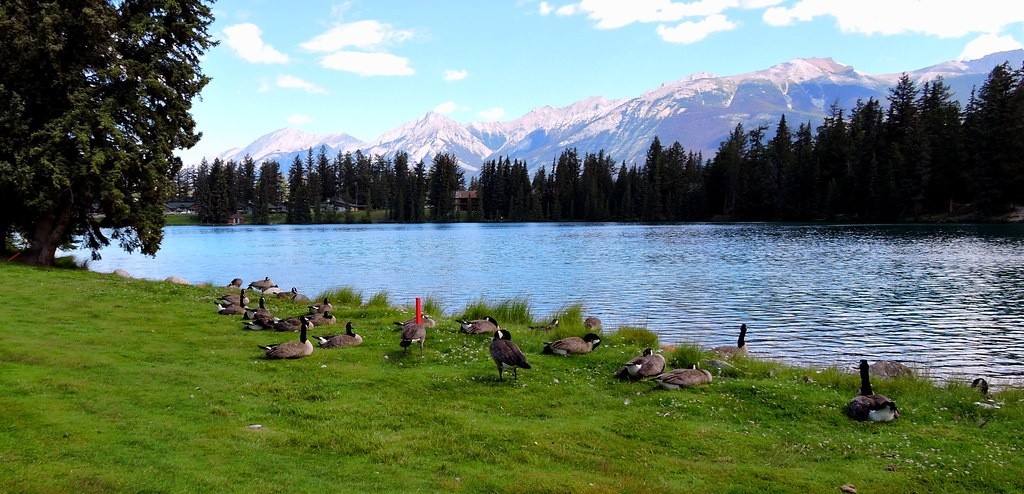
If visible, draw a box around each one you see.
[526,317,602,356]
[972,377,1002,409]
[457,316,531,382]
[393,298,436,357]
[843,359,900,422]
[215,277,363,359]
[611,322,750,394]
